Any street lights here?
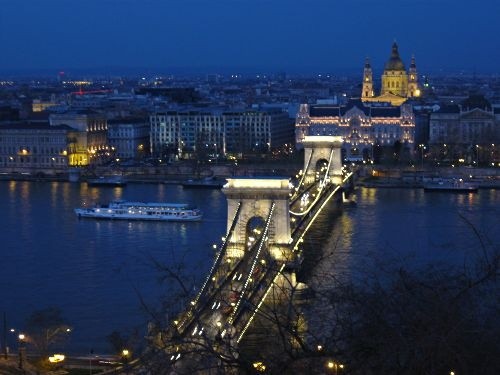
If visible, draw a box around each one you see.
[9,328,25,369]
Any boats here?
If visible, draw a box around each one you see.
[177,176,226,190]
[423,177,480,193]
[86,176,127,187]
[72,199,205,223]
